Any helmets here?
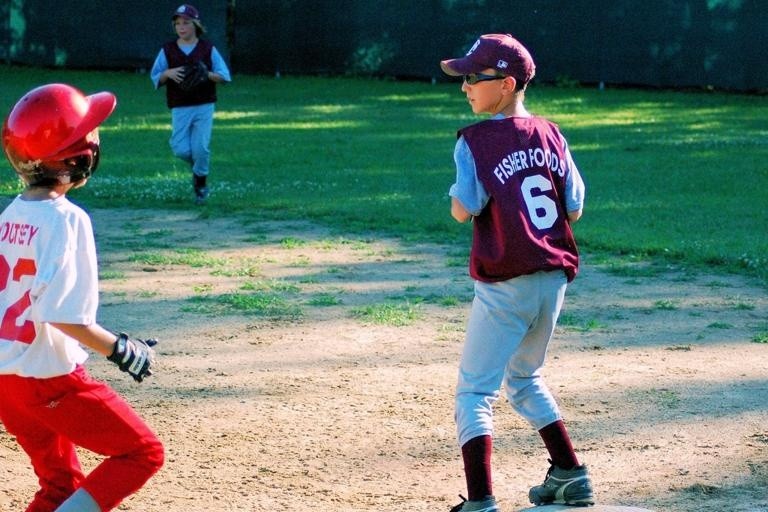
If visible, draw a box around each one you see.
[3,82,116,184]
[172,5,199,19]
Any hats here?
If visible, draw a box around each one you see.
[440,33,536,82]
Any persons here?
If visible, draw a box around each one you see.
[150,4,232,200]
[441,31,597,512]
[0,83,166,512]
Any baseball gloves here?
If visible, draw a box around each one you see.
[182,62,209,87]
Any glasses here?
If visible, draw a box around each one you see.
[461,73,504,84]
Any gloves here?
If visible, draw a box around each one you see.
[107,332,157,383]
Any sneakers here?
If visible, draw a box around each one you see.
[529,459,594,506]
[194,185,207,203]
[450,494,497,512]
